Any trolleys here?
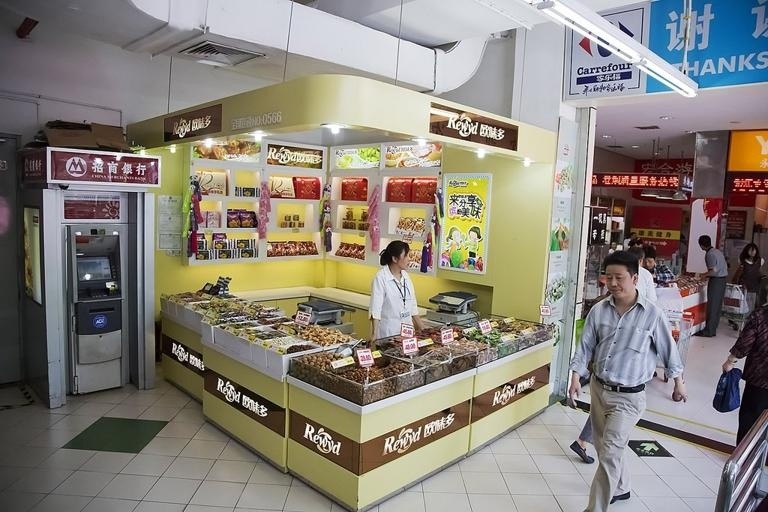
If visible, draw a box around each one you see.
[661,308,694,382]
[720,281,750,332]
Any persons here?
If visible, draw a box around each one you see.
[567,232,687,512]
[721,297,768,447]
[728,242,765,331]
[693,235,729,337]
[367,240,425,345]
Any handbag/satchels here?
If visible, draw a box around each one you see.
[729,286,749,314]
[712,368,743,413]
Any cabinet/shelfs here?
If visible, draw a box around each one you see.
[182,138,328,266]
[326,141,445,278]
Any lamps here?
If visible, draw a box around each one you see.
[656,145,672,200]
[640,140,656,198]
[672,151,688,201]
[536,0,699,98]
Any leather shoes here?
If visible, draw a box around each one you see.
[570,441,594,464]
[610,492,630,504]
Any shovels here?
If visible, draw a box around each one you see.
[333,337,364,357]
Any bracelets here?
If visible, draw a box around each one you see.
[727,357,738,364]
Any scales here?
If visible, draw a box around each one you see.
[288,300,355,336]
[427,290,482,326]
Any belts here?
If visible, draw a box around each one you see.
[594,372,646,393]
